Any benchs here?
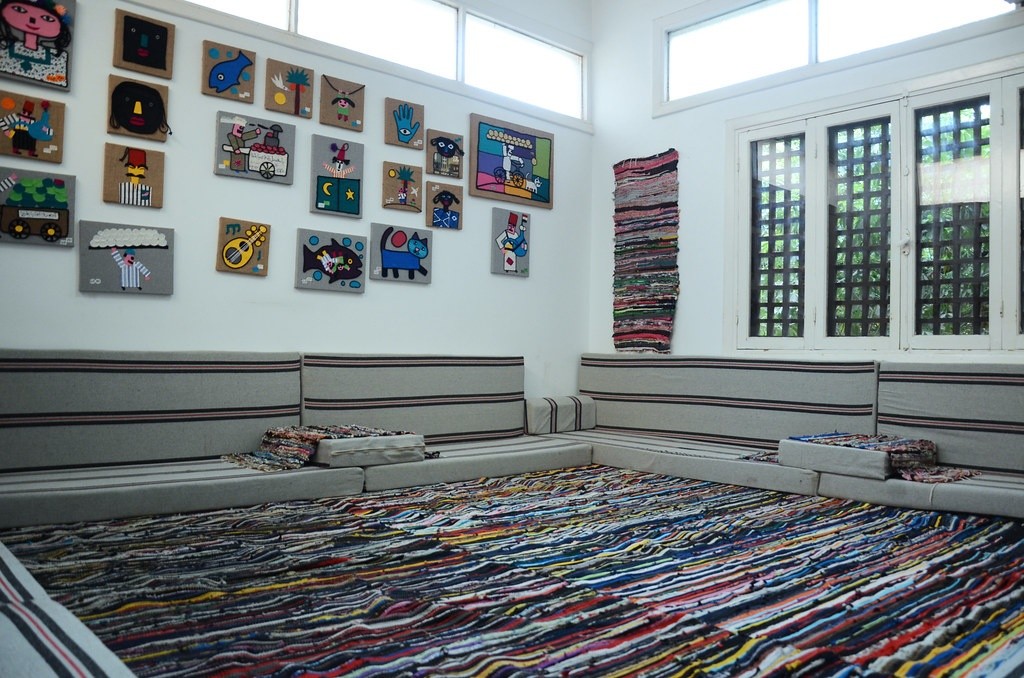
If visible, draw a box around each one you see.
[0,353,1024,520]
